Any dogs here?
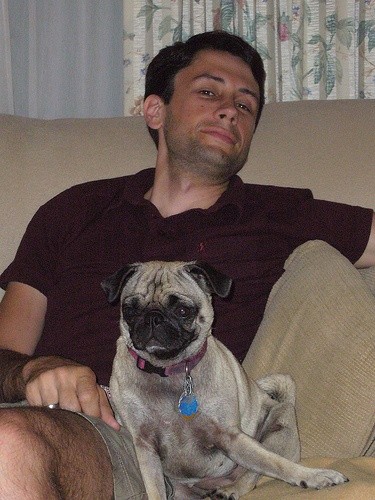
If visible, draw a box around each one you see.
[100,260,350,500]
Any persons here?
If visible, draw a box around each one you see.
[1,28,374,499]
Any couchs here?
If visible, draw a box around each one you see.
[0,98,375,302]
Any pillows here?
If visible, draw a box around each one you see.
[241,240,375,487]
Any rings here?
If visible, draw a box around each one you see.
[43,403,60,410]
